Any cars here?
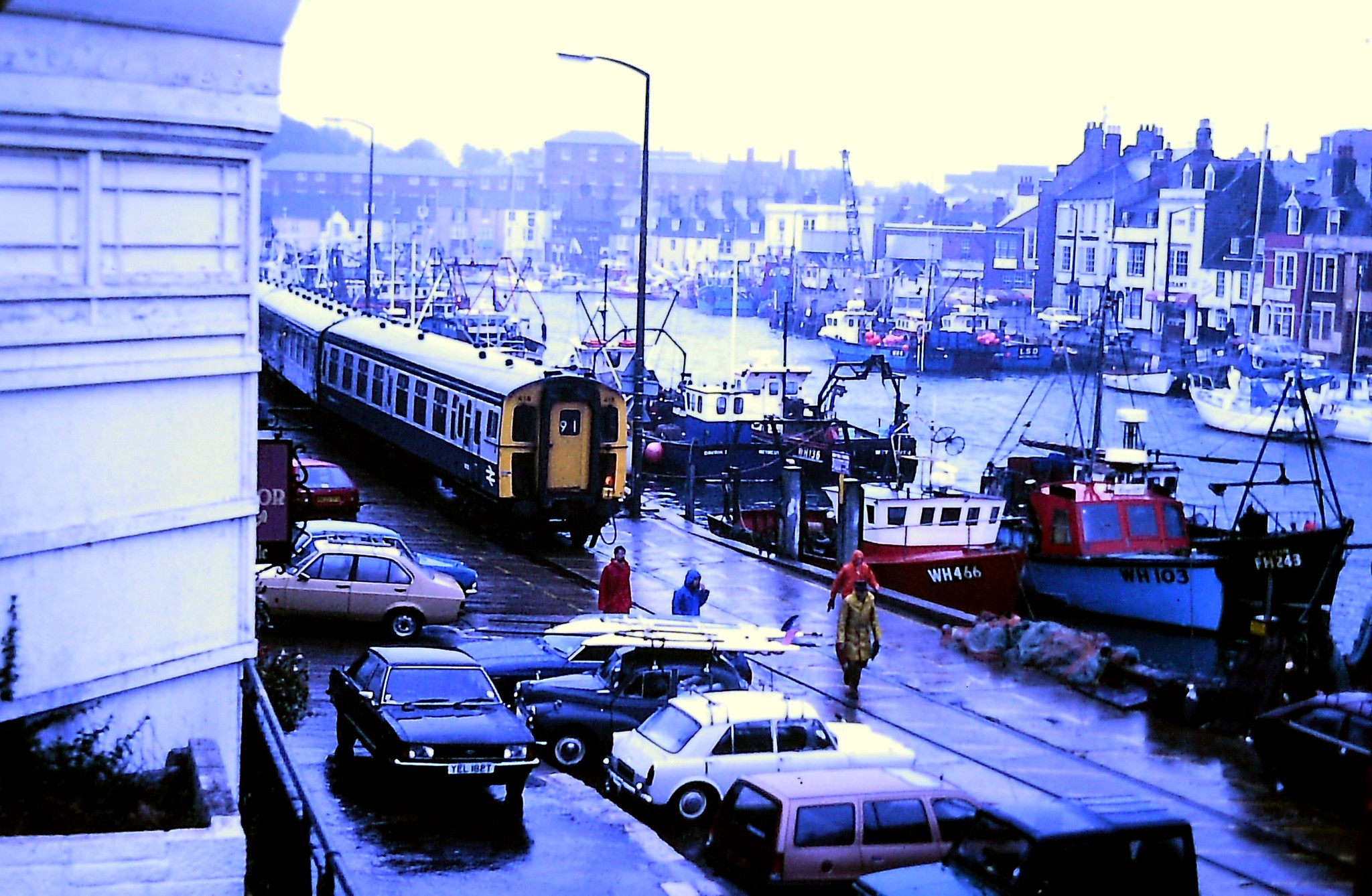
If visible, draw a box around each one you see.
[256,457,544,642]
[324,647,542,791]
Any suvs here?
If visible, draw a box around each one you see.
[456,631,1200,896]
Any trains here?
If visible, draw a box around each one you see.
[258,276,628,548]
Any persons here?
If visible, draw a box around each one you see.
[825,551,880,612]
[836,580,881,698]
[673,569,710,618]
[598,545,633,616]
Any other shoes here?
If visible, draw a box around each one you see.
[849,684,859,700]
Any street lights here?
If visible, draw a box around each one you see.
[324,116,373,314]
[556,52,650,518]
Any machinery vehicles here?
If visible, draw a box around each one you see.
[839,145,866,271]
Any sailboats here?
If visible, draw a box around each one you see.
[1186,123,1339,443]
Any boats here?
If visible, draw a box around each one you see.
[297,246,1372,634]
